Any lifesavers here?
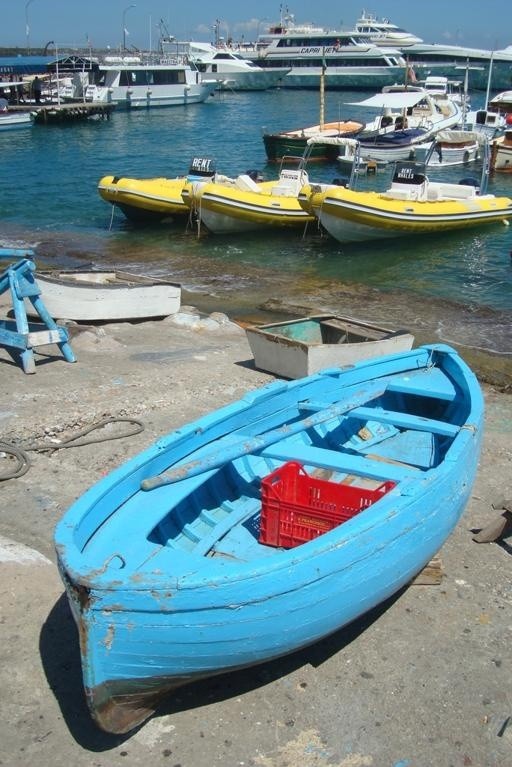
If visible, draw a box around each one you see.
[335,43,340,49]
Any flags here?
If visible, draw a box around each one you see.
[409,67,418,84]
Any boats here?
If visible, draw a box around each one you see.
[264,87,512,173]
[51,342,482,733]
